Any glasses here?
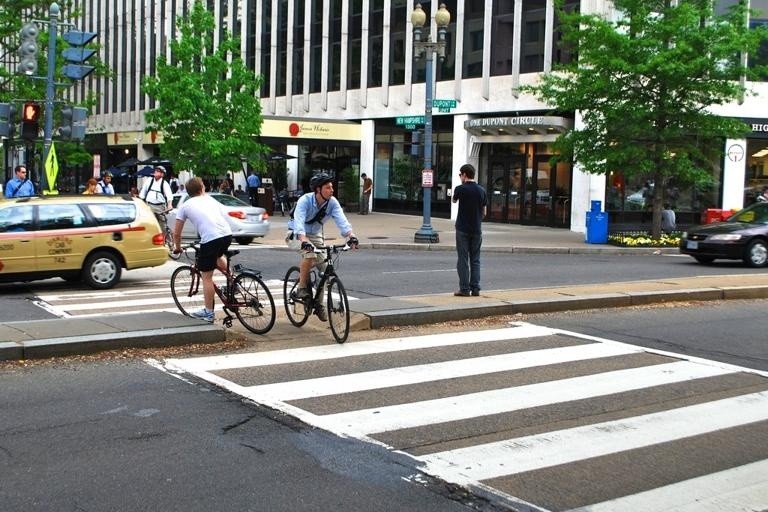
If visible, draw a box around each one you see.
[22,171,26,173]
[458,173,462,176]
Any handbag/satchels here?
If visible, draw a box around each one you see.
[288,200,330,225]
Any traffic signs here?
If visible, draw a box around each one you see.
[433,100,456,108]
[439,108,450,113]
[405,125,416,129]
[396,117,425,125]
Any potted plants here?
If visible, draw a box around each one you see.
[339,168,358,212]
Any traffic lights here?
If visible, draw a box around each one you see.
[19,23,38,76]
[21,104,41,141]
[64,31,97,80]
[1,103,16,139]
[59,105,88,142]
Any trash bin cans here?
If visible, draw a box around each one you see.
[701,208,755,225]
[586,200,608,244]
[257,185,273,216]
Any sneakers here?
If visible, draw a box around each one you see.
[315,306,327,322]
[189,309,215,323]
[296,288,309,298]
[221,285,241,295]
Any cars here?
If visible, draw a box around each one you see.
[627,176,768,209]
[165,192,269,245]
[389,154,550,218]
[0,194,169,290]
[679,201,768,268]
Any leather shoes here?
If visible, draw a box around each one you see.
[471,289,478,296]
[453,291,469,296]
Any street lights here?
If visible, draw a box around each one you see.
[411,3,451,242]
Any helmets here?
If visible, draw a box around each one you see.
[309,172,334,192]
[154,165,166,175]
[102,170,113,178]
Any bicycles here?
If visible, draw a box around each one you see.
[283,240,358,344]
[170,239,276,334]
[154,208,181,260]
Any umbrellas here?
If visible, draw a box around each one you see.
[265,153,297,160]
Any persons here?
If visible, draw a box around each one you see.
[81,178,98,195]
[174,177,234,324]
[286,172,359,322]
[359,173,373,215]
[4,166,36,197]
[662,201,676,231]
[451,164,488,296]
[138,165,175,252]
[38,158,267,208]
[95,172,116,196]
